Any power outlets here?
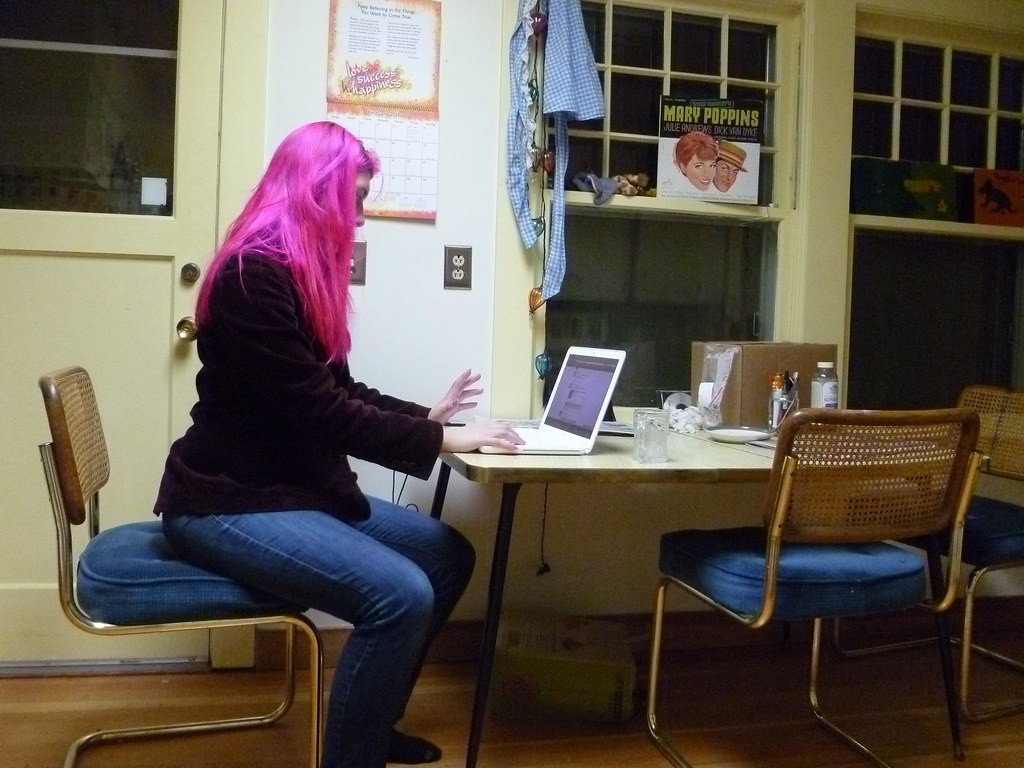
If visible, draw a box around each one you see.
[443,245,472,290]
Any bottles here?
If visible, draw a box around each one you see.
[810,361,839,425]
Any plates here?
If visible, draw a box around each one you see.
[706,425,771,443]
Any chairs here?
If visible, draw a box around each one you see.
[646,408,983,768]
[38,364,324,768]
[831,384,1024,724]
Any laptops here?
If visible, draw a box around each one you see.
[478,347,626,454]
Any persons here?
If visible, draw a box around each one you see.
[158,120,528,768]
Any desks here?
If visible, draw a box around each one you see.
[429,422,990,768]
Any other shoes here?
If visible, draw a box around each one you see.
[387,730,442,764]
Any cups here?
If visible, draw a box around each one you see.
[634,409,671,464]
[769,390,799,435]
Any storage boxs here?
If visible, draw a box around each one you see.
[691,341,838,429]
